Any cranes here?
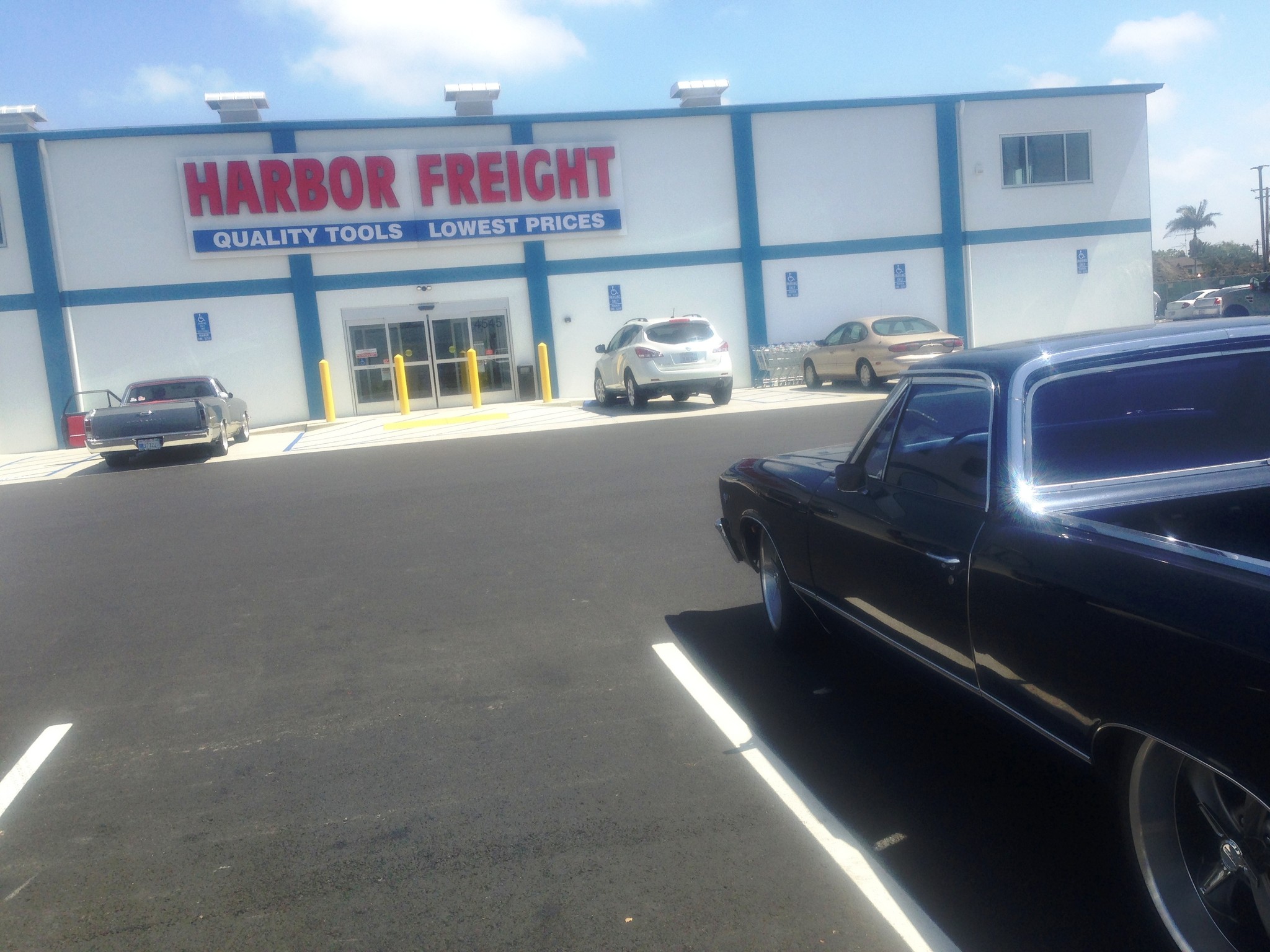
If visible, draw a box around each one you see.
[1167,231,1205,255]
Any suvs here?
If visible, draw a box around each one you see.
[594,314,733,409]
[1218,273,1270,317]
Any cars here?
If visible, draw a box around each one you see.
[1164,289,1220,322]
[1193,284,1250,318]
[800,315,964,390]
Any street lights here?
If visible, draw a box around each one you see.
[1250,165,1270,273]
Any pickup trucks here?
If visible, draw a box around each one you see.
[60,376,250,467]
[714,315,1270,952]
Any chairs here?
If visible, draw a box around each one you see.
[195,385,211,397]
[153,387,166,400]
